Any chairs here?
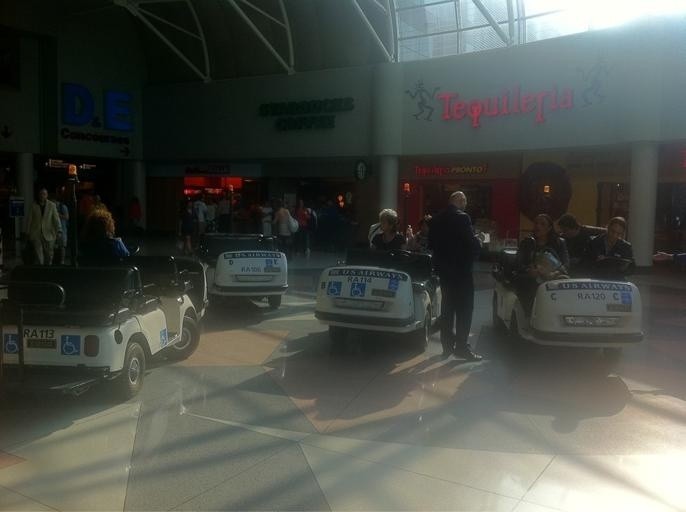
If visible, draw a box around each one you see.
[123,255,193,297]
[77,229,119,265]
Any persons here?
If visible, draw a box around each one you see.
[367,209,404,255]
[510,214,574,302]
[652,250,686,264]
[558,214,607,275]
[428,191,486,362]
[23,186,359,267]
[405,213,434,258]
[520,235,567,283]
[581,215,636,281]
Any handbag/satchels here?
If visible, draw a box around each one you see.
[287,210,300,233]
[20,244,36,266]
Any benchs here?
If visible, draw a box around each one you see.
[0,264,158,325]
[201,233,279,265]
[337,246,440,291]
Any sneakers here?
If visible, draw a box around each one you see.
[442,344,483,364]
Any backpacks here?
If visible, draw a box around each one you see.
[304,207,319,232]
